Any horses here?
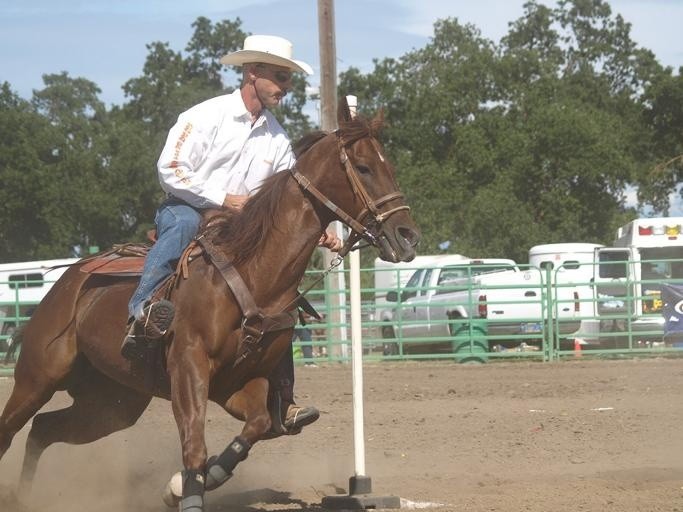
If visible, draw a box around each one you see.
[0,94,420,512]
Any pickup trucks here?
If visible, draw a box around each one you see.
[374,255,583,357]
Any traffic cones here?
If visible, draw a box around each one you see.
[572,336,583,362]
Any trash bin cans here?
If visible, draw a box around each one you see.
[451,318,489,363]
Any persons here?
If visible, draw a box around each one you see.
[290,312,324,368]
[115,33,342,440]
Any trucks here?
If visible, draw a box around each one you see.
[591,214,682,355]
[525,239,626,351]
[367,251,472,329]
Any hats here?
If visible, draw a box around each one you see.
[219,35,314,76]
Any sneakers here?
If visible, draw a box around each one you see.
[280,399,320,435]
[120,299,176,361]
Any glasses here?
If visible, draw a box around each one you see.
[255,64,292,83]
